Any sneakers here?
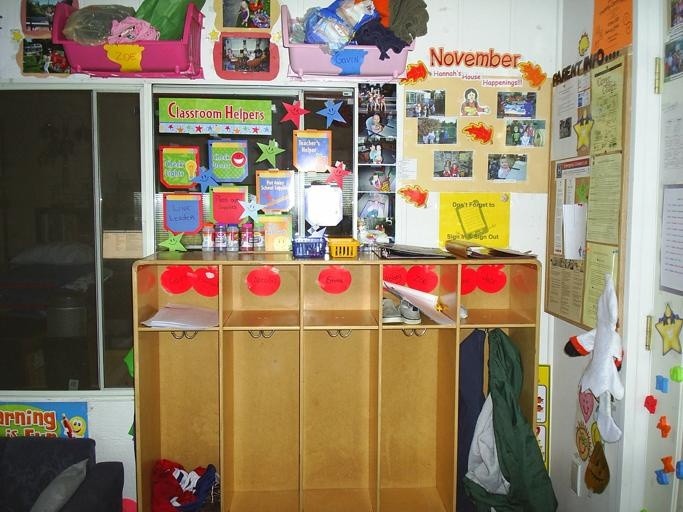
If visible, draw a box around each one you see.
[382,297,421,325]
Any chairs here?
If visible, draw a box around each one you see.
[0,433,127,511]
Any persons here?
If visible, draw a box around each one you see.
[414,101,459,178]
[227,45,262,62]
[498,95,536,179]
[365,88,385,165]
[668,50,680,75]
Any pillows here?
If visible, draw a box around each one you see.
[27,454,89,511]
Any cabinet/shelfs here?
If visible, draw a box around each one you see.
[131,245,550,508]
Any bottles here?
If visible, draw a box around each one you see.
[201,222,265,252]
